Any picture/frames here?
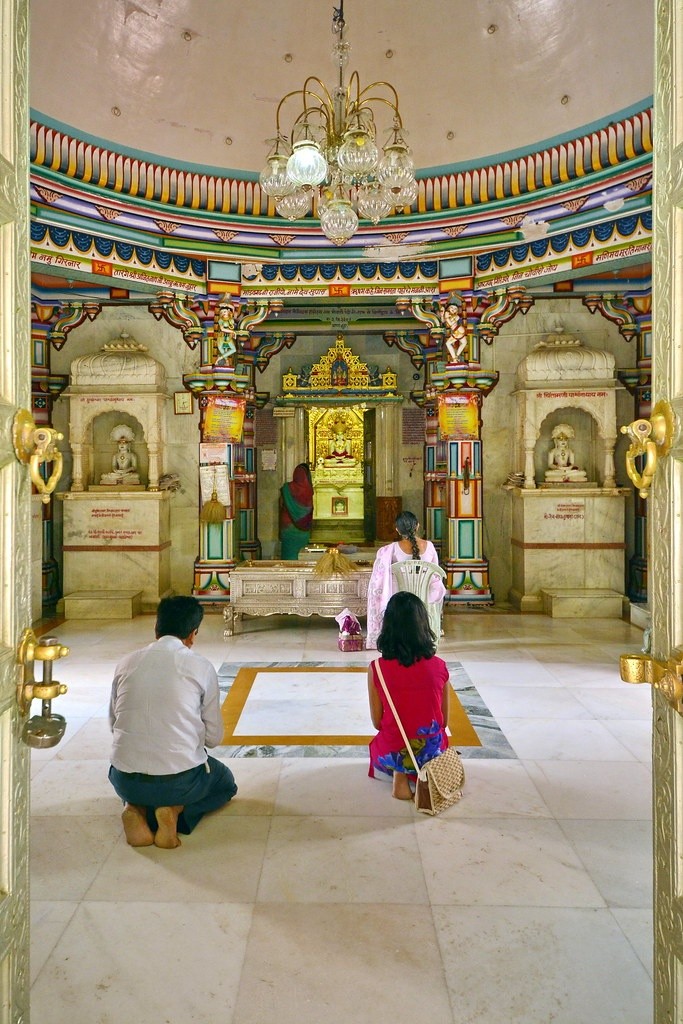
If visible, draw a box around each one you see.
[173,391,194,415]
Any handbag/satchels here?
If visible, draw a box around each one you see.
[415,748,464,814]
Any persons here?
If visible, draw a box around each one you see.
[369,591,450,801]
[324,418,356,463]
[214,292,237,366]
[108,595,239,849]
[101,424,141,479]
[366,509,447,650]
[545,424,587,479]
[440,303,467,364]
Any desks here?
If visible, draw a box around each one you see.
[298,546,382,559]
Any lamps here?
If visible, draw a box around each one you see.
[257,0,418,247]
